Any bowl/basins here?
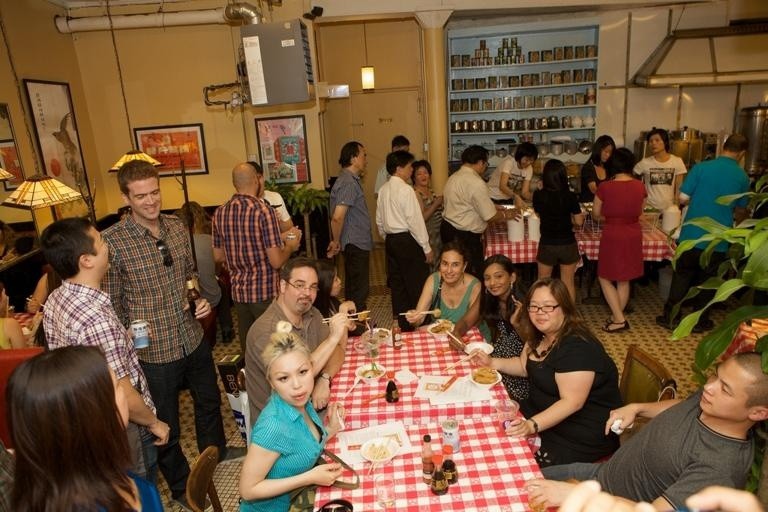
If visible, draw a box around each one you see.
[365,328,389,340]
[360,438,400,465]
[469,370,502,389]
[426,322,454,337]
[355,364,386,384]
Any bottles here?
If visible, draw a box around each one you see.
[422,434,458,496]
[387,370,400,403]
[391,317,402,350]
[185,276,201,321]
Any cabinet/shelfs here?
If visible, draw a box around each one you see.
[447,19,600,163]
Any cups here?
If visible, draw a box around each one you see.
[494,399,520,426]
[528,483,544,512]
[374,474,396,509]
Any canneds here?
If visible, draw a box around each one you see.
[131,319,150,348]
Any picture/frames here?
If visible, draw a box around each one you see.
[133,123,208,177]
[23,79,96,222]
[254,115,311,186]
[0,139,24,192]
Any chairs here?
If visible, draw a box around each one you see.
[185,445,224,512]
[237,367,246,392]
[592,344,675,464]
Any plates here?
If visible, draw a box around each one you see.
[463,342,494,355]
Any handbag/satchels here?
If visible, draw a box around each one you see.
[288,449,359,512]
[619,416,652,446]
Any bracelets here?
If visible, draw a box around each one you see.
[528,418,538,434]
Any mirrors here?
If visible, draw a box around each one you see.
[0,101,42,273]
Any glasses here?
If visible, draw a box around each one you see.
[156,240,173,267]
[321,507,352,512]
[526,303,560,312]
[284,279,320,291]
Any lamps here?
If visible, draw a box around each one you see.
[0,24,83,212]
[360,24,375,93]
[106,2,163,173]
[0,167,15,181]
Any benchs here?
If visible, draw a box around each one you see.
[0,348,47,449]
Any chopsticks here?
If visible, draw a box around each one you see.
[399,309,434,316]
[342,375,361,398]
[322,311,372,322]
[444,350,481,370]
[367,438,390,477]
[26,297,44,307]
[445,329,467,347]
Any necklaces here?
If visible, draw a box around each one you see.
[531,333,557,358]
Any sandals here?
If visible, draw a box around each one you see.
[602,316,629,332]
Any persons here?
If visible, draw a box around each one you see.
[374,135,583,332]
[524,351,768,512]
[99,159,247,512]
[449,255,531,402]
[41,217,171,485]
[6,345,165,512]
[245,256,345,430]
[180,160,302,351]
[470,277,624,469]
[325,142,373,313]
[0,220,36,264]
[0,268,43,348]
[579,131,750,333]
[238,332,346,512]
[557,480,766,512]
[25,272,48,313]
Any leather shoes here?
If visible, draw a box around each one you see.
[656,316,680,329]
[692,320,713,333]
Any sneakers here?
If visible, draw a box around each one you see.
[218,447,247,461]
[172,490,213,512]
[209,328,235,347]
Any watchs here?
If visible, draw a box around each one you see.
[318,372,332,382]
